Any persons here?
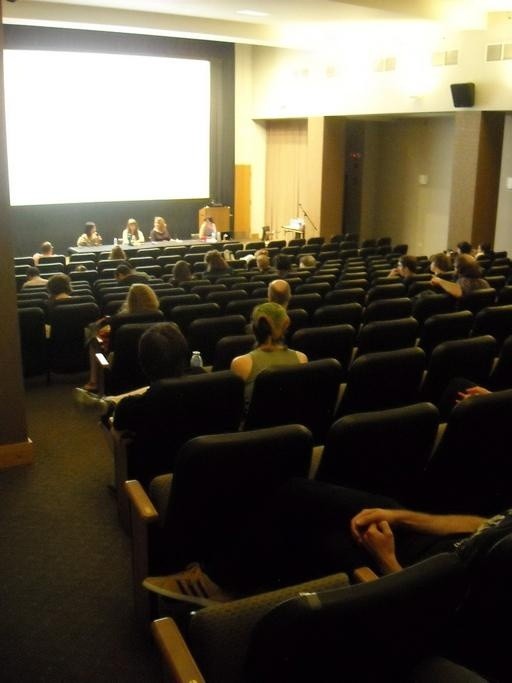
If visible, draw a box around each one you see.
[22,213,316,338]
[444,375,502,407]
[387,239,494,298]
[84,280,319,440]
[142,505,509,607]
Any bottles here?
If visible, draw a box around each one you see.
[113,238,118,245]
[189,350,202,369]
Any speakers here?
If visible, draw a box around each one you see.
[450,83,475,107]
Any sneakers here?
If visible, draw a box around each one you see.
[141,563,238,607]
[73,387,108,417]
